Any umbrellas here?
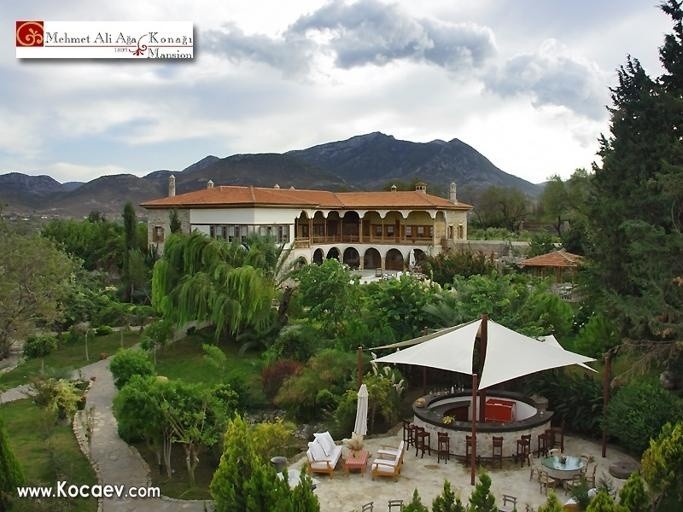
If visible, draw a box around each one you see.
[353,382,369,437]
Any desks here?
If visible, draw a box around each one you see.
[539,455,584,482]
[341,443,370,478]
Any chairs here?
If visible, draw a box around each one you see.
[348,495,532,511]
[399,420,565,466]
[585,465,597,488]
[379,439,407,464]
[564,474,580,497]
[528,455,540,481]
[539,471,555,496]
[371,450,397,482]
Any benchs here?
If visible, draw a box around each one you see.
[305,432,342,479]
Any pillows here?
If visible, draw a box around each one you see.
[312,432,336,456]
[307,439,325,462]
